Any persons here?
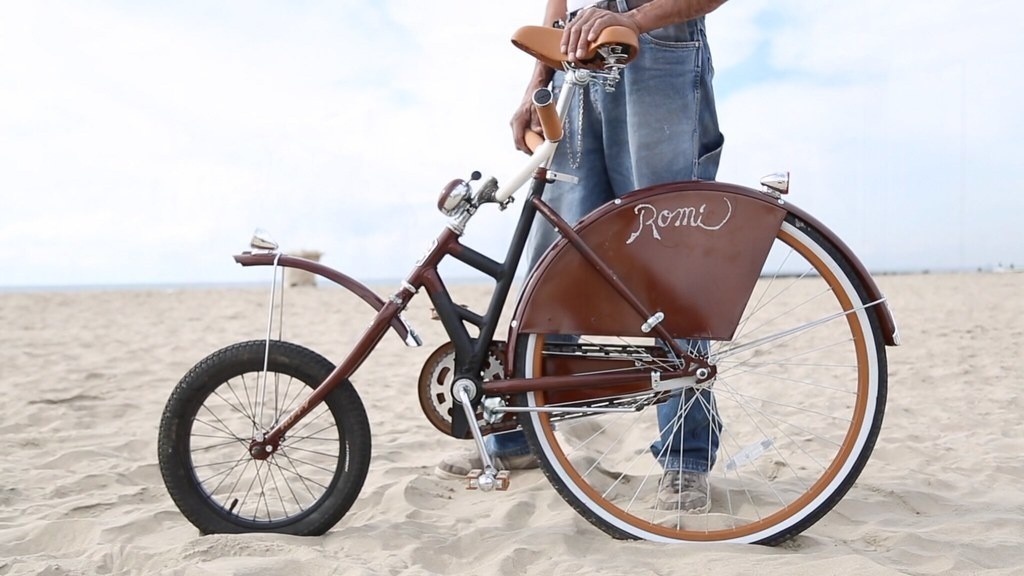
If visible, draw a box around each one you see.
[432,0,725,510]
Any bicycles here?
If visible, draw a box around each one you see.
[155,26,900,547]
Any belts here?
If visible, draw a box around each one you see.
[552,0,654,29]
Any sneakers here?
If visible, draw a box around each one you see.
[435,448,540,481]
[655,470,712,515]
